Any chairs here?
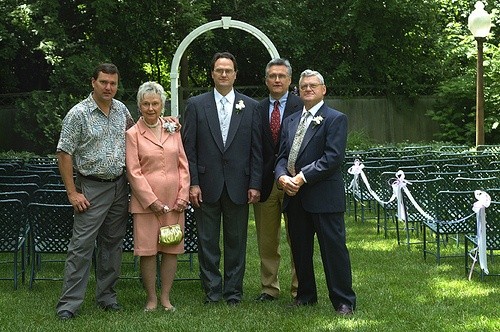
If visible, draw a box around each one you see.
[341,144,500,283]
[0,155,202,290]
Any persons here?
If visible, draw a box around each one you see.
[183,52,263,304]
[124,81,190,315]
[274,70,357,315]
[57,63,182,321]
[253,59,304,301]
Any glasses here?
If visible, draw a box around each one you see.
[268,73,290,79]
[299,83,323,90]
[213,68,235,76]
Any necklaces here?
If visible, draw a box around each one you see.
[142,116,159,128]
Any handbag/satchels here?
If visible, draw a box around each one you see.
[158,210,183,247]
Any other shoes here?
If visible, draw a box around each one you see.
[164,306,175,312]
[337,305,352,315]
[296,301,318,306]
[144,307,156,312]
[204,301,217,305]
[227,299,240,307]
[255,293,279,302]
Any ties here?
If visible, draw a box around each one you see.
[219,97,230,148]
[271,100,280,148]
[287,113,310,176]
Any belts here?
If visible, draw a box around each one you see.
[77,171,124,182]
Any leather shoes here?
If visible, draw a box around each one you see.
[57,310,73,321]
[102,304,124,312]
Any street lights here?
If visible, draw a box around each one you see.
[467,2,491,150]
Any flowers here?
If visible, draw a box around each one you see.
[163,122,179,133]
[234,99,246,114]
[311,115,324,128]
